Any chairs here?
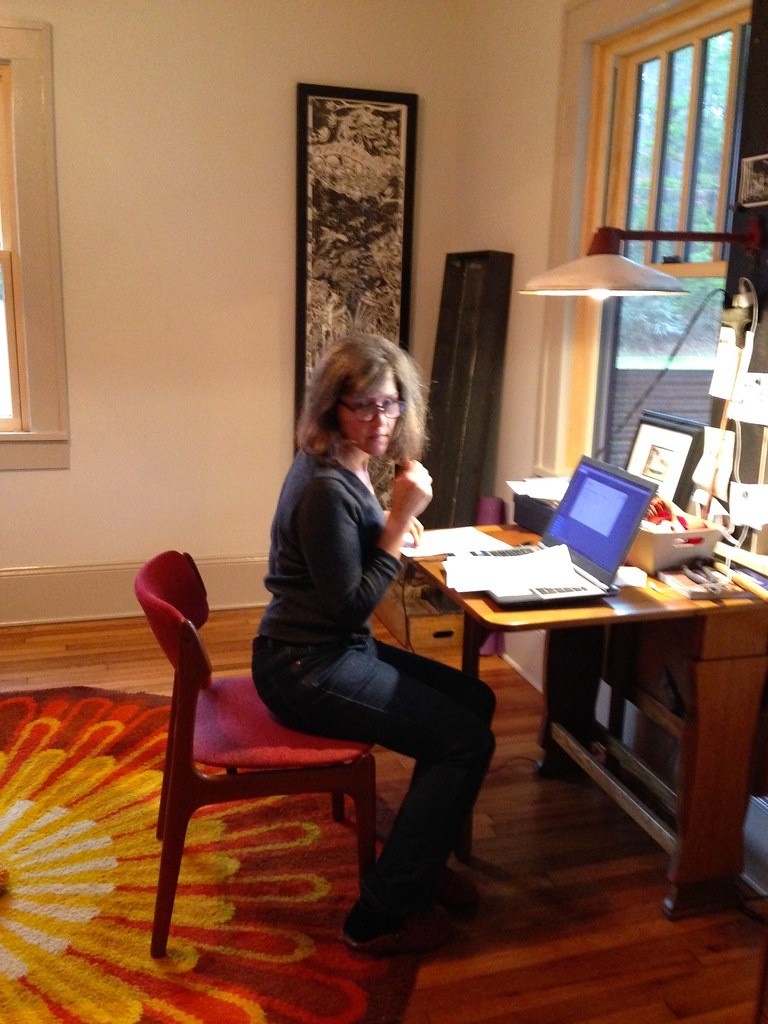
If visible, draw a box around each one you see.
[134,551,376,960]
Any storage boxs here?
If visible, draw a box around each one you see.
[625,512,723,577]
[513,493,556,537]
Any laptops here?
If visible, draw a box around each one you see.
[451,454,658,605]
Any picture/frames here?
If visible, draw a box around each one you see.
[293,83,420,514]
[624,409,704,510]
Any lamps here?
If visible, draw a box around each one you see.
[515,218,765,302]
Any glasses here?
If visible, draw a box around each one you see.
[341,399,406,422]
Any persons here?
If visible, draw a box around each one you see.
[250,333,502,952]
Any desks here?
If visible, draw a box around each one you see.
[400,524,768,919]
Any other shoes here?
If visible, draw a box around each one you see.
[436,866,481,910]
[341,904,454,955]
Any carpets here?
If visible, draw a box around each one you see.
[1,686,427,1024]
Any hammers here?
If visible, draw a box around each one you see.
[697,555,768,604]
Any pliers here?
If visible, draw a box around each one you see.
[680,560,729,594]
